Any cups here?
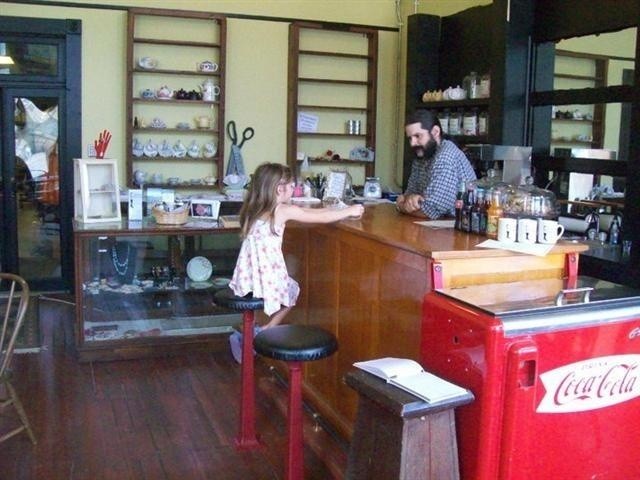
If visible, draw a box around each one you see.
[496,217,565,245]
[622,240,632,252]
[346,120,361,135]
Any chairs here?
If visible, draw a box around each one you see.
[0,272,38,453]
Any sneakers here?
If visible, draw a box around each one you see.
[229,331,242,365]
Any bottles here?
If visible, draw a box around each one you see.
[430,105,490,136]
[133,115,138,129]
[462,70,492,100]
[454,180,505,240]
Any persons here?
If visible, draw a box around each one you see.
[229,163,366,364]
[396,110,478,219]
[193,204,212,217]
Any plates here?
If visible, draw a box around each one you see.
[186,256,214,282]
[190,282,213,289]
[214,278,231,287]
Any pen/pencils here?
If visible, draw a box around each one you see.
[307,174,327,198]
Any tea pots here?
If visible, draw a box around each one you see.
[136,55,160,70]
[198,78,220,101]
[576,133,589,142]
[196,60,218,72]
[555,110,592,121]
[422,85,467,102]
[130,138,217,159]
[139,84,201,100]
[134,168,218,186]
[194,115,214,128]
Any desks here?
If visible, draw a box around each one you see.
[342,365,477,479]
[115,192,247,217]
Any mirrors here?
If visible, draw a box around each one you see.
[530,23,638,273]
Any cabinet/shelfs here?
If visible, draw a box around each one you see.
[548,49,610,150]
[70,212,245,364]
[72,157,122,223]
[126,7,226,191]
[414,96,494,145]
[288,21,379,181]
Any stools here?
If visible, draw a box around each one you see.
[209,288,270,451]
[252,323,338,479]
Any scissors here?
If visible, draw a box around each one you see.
[227,120,254,148]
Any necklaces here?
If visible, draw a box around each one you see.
[112,244,130,276]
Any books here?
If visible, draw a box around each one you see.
[352,357,468,404]
